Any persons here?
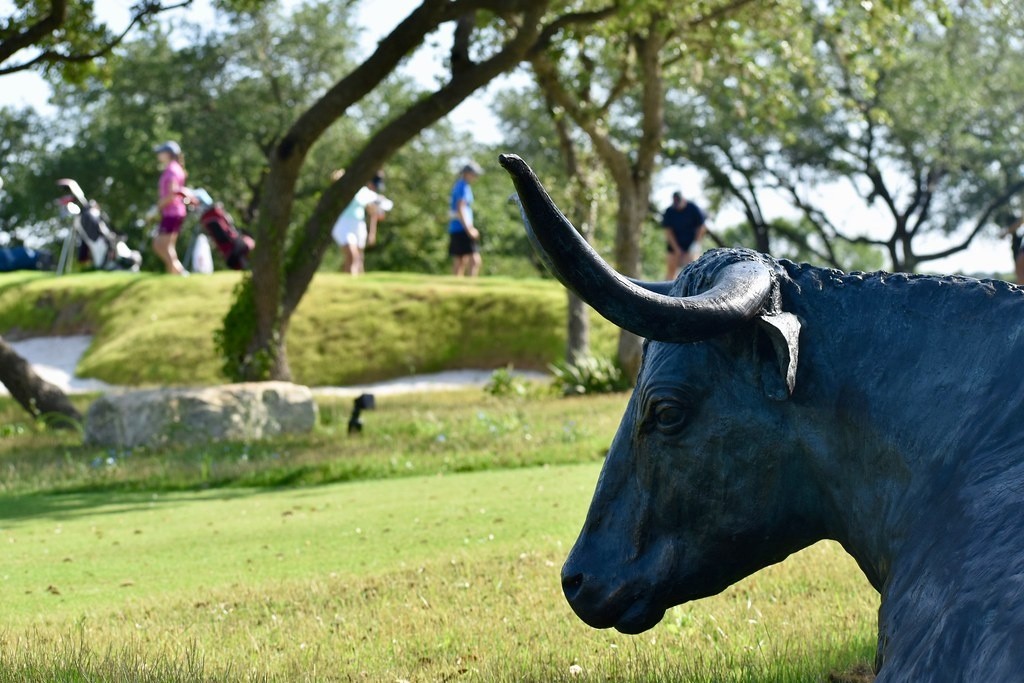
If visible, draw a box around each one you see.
[331,168,394,275]
[660,191,710,280]
[152,141,197,277]
[448,161,483,277]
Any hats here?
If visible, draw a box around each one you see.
[462,161,486,175]
[373,170,386,191]
[153,140,181,155]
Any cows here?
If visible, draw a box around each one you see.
[497,153,1024,683]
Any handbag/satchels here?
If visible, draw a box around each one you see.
[0,246,42,272]
